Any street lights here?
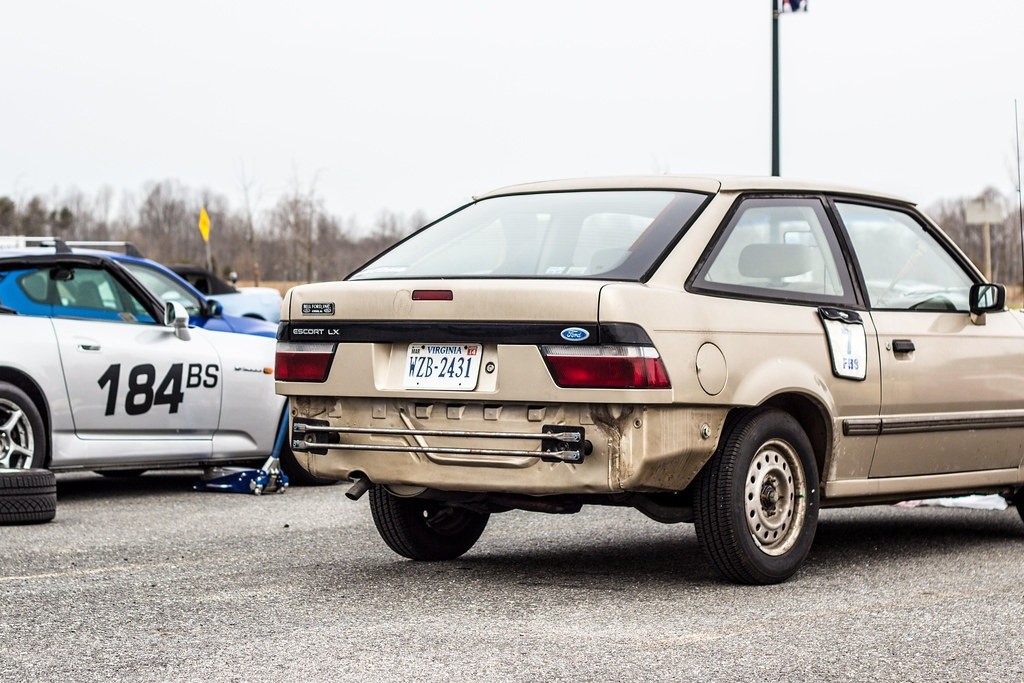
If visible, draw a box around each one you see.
[771,0,810,180]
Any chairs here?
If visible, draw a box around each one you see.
[74,281,105,309]
[23,274,45,302]
[592,246,628,271]
[741,243,836,295]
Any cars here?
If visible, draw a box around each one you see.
[270,177,1024,586]
[0,236,343,491]
